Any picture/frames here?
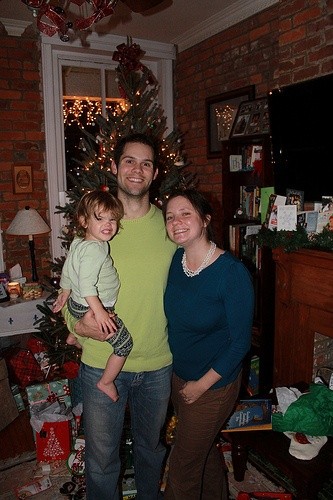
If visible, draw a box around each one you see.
[229,96,270,137]
[205,83,256,160]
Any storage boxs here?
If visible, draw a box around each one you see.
[0,339,85,464]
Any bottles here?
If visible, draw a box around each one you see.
[231,441,246,482]
[8,282,20,299]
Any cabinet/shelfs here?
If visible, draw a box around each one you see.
[222,136,275,356]
[272,247,333,389]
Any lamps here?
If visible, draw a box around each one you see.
[6,206,52,281]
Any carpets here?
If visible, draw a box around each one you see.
[0,436,283,500]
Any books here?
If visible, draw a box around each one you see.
[226,143,275,272]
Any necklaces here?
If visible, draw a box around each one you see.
[181,241,216,276]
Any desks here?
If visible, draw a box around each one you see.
[232,429,333,500]
[0,281,56,337]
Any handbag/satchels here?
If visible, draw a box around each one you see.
[36,419,72,462]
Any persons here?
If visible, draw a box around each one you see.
[162,188,255,500]
[53,190,133,403]
[59,132,179,500]
